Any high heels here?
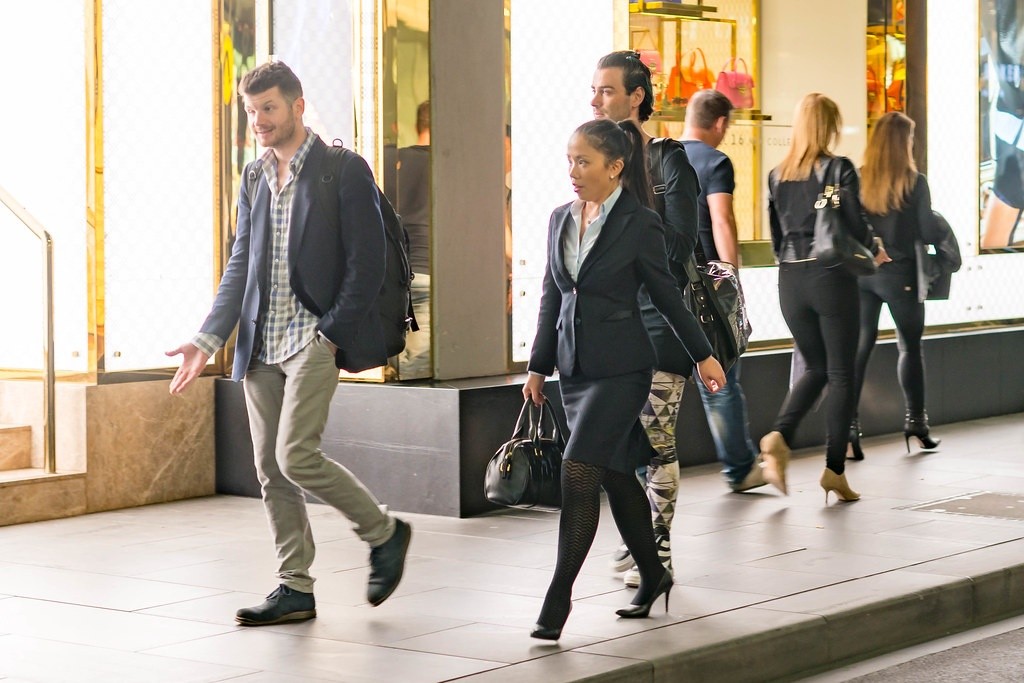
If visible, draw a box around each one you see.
[530,599,571,642]
[902,410,941,454]
[821,465,860,504]
[846,424,865,461]
[617,569,673,619]
[757,432,790,496]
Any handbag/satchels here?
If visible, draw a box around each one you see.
[714,58,755,110]
[485,393,571,511]
[867,65,905,115]
[682,261,753,377]
[813,158,880,274]
[664,47,713,104]
[635,31,662,75]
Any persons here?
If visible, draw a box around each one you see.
[590,49,701,587]
[522,120,727,640]
[166,59,413,628]
[676,90,772,493]
[982,0,1024,248]
[857,113,944,460]
[757,92,892,506]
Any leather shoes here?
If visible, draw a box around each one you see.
[236,590,318,626]
[369,518,411,606]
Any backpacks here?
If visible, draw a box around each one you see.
[244,146,413,357]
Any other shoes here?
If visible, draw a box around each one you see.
[730,461,768,492]
[615,544,642,588]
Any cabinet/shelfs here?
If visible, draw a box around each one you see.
[628,0,773,241]
